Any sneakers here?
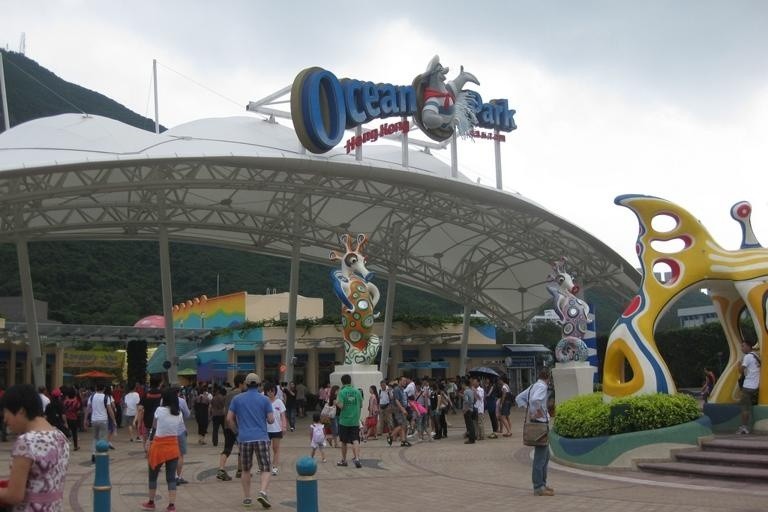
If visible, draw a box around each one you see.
[216,470,232,480]
[401,441,412,447]
[464,440,474,444]
[176,481,179,486]
[431,432,436,436]
[176,477,189,484]
[488,433,498,439]
[736,425,751,435]
[387,436,393,447]
[166,506,176,512]
[141,503,155,510]
[130,438,134,441]
[442,435,447,438]
[322,458,327,463]
[336,459,348,466]
[503,432,513,437]
[545,485,554,491]
[534,490,554,496]
[429,438,435,442]
[272,465,278,476]
[241,497,252,507]
[198,438,202,444]
[108,442,115,450]
[92,453,96,462]
[417,438,423,442]
[407,434,413,438]
[413,431,418,436]
[432,436,440,440]
[135,438,142,442]
[74,446,80,451]
[355,459,361,469]
[203,440,208,445]
[290,427,294,432]
[257,493,272,508]
[235,471,253,478]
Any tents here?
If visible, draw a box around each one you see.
[399,361,451,368]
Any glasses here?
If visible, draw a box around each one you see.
[431,66,443,75]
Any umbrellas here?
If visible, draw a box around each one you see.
[75,371,115,377]
[469,367,499,387]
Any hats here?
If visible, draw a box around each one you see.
[245,373,261,384]
[421,55,440,78]
[51,388,63,397]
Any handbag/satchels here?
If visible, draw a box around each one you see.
[471,404,478,421]
[380,403,388,410]
[365,416,377,428]
[738,353,761,390]
[321,403,336,419]
[523,384,550,446]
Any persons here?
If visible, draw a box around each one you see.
[378,380,394,435]
[284,382,297,432]
[703,367,716,403]
[516,367,555,496]
[404,375,416,396]
[85,383,116,461]
[365,385,380,440]
[407,395,427,442]
[328,385,341,448]
[39,386,49,412]
[140,388,185,512]
[142,374,163,458]
[498,376,514,436]
[310,413,326,463]
[277,382,288,401]
[460,380,475,444]
[195,387,210,444]
[44,388,67,431]
[737,342,762,434]
[103,386,116,449]
[114,384,123,428]
[407,381,428,437]
[296,379,306,417]
[212,388,226,446]
[263,383,286,476]
[216,375,248,481]
[227,373,274,509]
[421,379,433,407]
[333,375,363,468]
[430,376,460,440]
[485,375,499,438]
[472,379,485,439]
[0,385,69,512]
[387,376,411,446]
[64,389,80,450]
[160,384,191,485]
[124,384,142,443]
